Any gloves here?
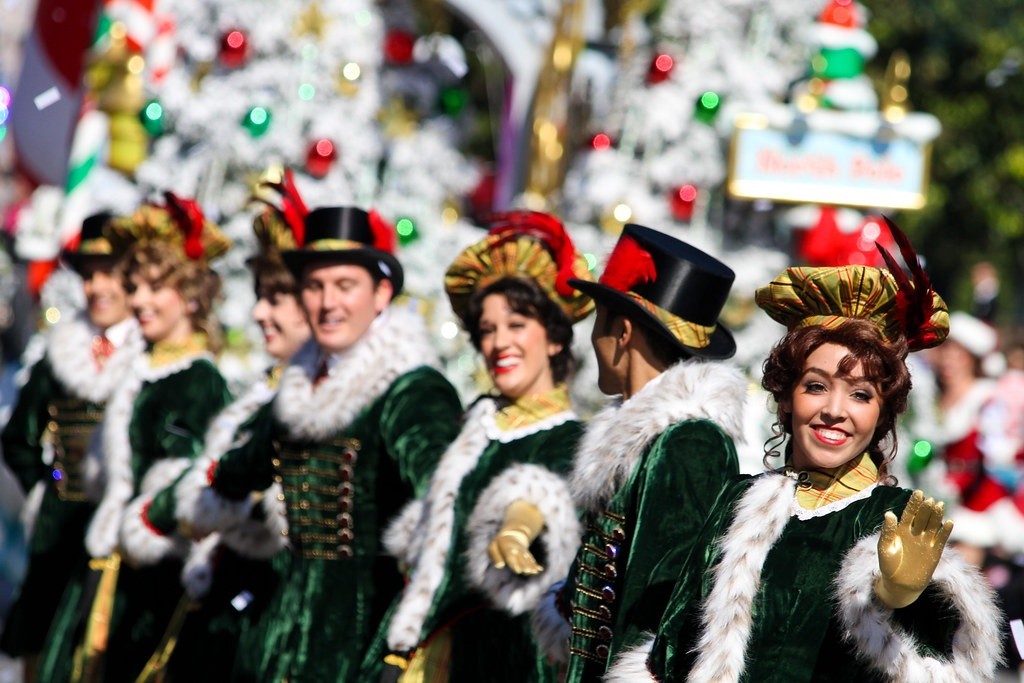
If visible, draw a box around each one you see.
[487,500,545,575]
[875,490,954,608]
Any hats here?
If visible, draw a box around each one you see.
[58,212,137,275]
[567,224,737,360]
[244,208,308,270]
[755,264,949,360]
[278,206,404,304]
[444,212,597,328]
[112,191,233,273]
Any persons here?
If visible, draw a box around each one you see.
[0,207,466,683]
[911,311,1024,683]
[529,224,746,683]
[615,264,1001,683]
[361,232,597,683]
